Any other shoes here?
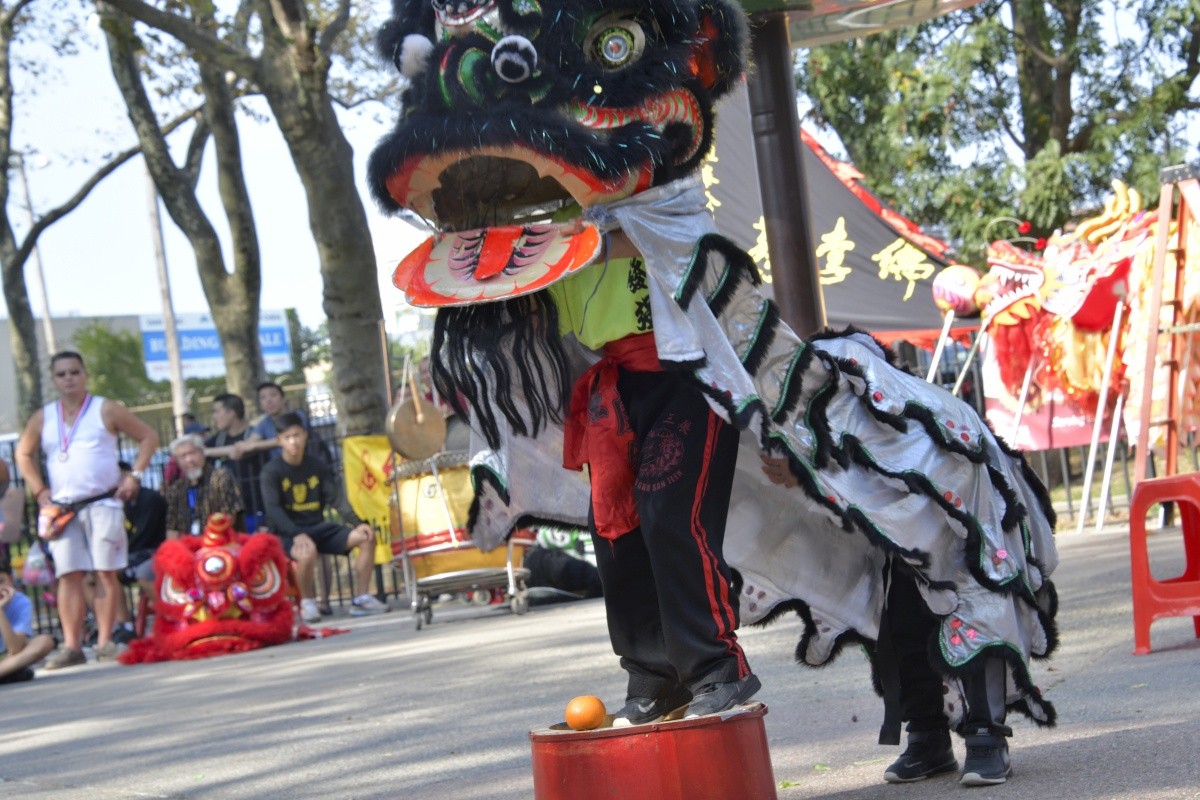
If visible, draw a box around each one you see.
[320,603,332,615]
[82,622,136,650]
[0,666,33,685]
[437,594,452,602]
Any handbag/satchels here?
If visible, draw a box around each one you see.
[0,488,26,544]
[524,547,604,607]
[37,490,117,540]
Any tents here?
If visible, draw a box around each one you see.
[703,58,988,420]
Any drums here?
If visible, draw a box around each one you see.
[384,450,525,597]
[383,398,447,461]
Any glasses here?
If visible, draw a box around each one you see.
[56,369,80,377]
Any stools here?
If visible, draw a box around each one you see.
[316,553,400,610]
[134,589,155,638]
[1130,471,1200,655]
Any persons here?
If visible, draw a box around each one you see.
[541,228,764,726]
[261,414,393,623]
[761,453,1014,784]
[109,461,166,635]
[0,456,146,645]
[15,351,161,670]
[165,383,346,615]
[166,433,252,545]
[0,561,57,685]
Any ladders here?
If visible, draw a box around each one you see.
[1130,163,1200,497]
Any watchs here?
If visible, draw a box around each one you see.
[125,470,144,478]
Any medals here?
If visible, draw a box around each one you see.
[58,451,68,462]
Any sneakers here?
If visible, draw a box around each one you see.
[613,680,693,727]
[349,595,392,615]
[884,727,959,781]
[96,642,128,661]
[46,647,86,669]
[683,671,762,720]
[300,598,322,621]
[959,721,1012,785]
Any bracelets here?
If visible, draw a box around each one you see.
[33,486,48,503]
